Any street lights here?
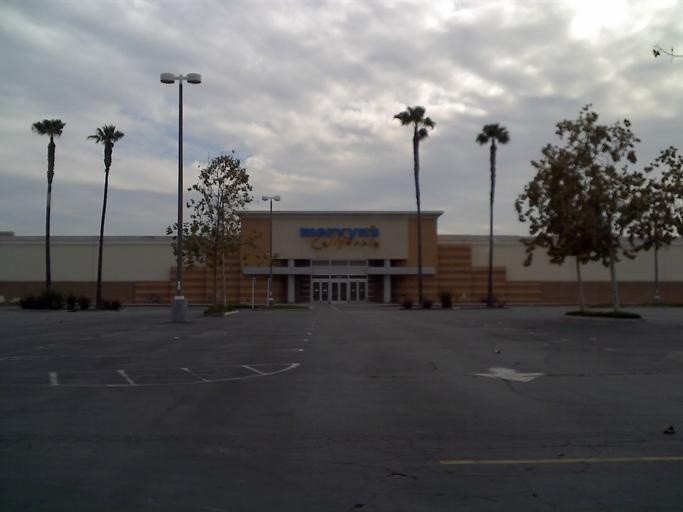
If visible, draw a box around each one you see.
[159,72,202,322]
[261,196,281,310]
